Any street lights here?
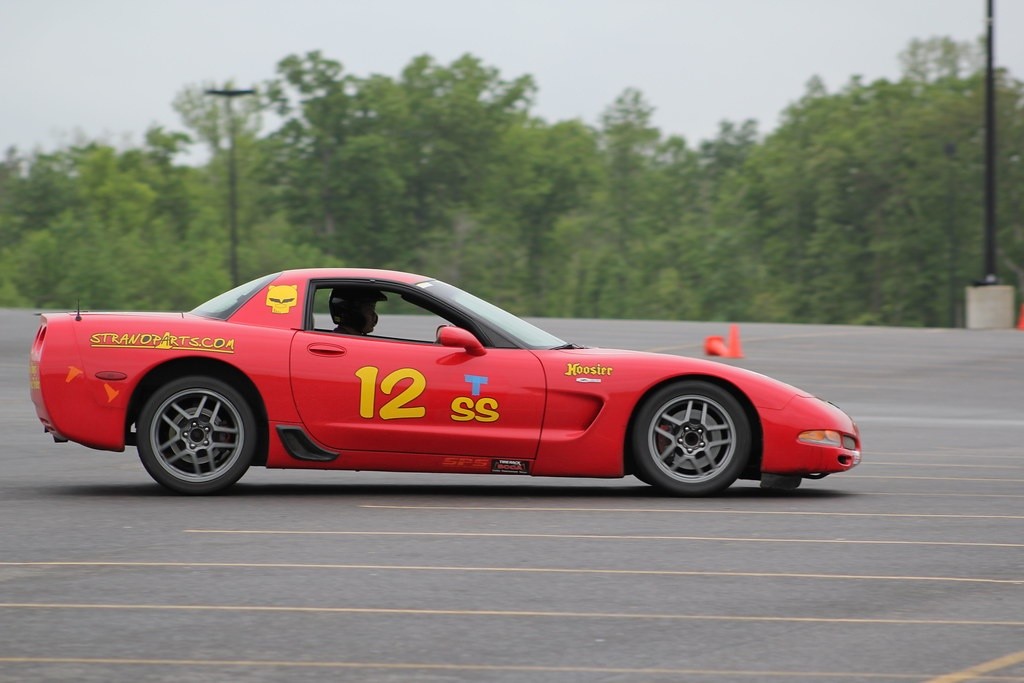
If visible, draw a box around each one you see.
[206,86,258,288]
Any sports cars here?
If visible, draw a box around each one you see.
[26,268,860,500]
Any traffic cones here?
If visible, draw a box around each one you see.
[722,321,745,361]
[1016,303,1024,330]
[707,335,728,361]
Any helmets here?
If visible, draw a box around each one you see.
[329,285,388,333]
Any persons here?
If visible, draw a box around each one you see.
[327,287,386,337]
